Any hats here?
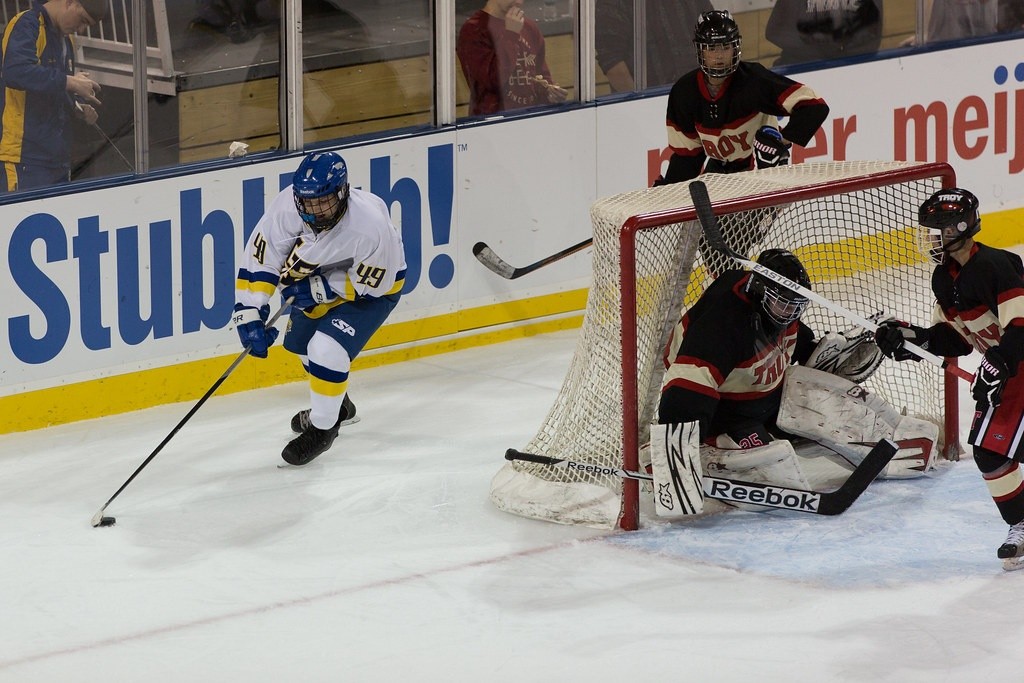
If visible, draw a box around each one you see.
[77,0,110,24]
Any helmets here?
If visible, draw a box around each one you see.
[693,9,743,79]
[291,151,349,231]
[916,188,981,265]
[746,248,813,331]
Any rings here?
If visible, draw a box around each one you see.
[91,108,96,111]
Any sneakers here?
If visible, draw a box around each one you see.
[291,390,360,434]
[997,519,1024,571]
[277,417,342,468]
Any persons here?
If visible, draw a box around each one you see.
[650,246,939,527]
[456,0,568,120]
[595,0,715,94]
[234,151,408,470]
[765,0,883,67]
[876,186,1024,570]
[897,0,1024,49]
[0,0,101,195]
[652,11,831,288]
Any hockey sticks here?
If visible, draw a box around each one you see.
[71,98,134,171]
[503,437,900,518]
[688,179,975,383]
[471,237,592,282]
[90,294,297,529]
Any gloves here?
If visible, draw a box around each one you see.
[971,344,1018,407]
[232,302,280,359]
[876,317,930,363]
[281,273,339,308]
[754,126,790,168]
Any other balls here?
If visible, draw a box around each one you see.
[100,517,115,526]
